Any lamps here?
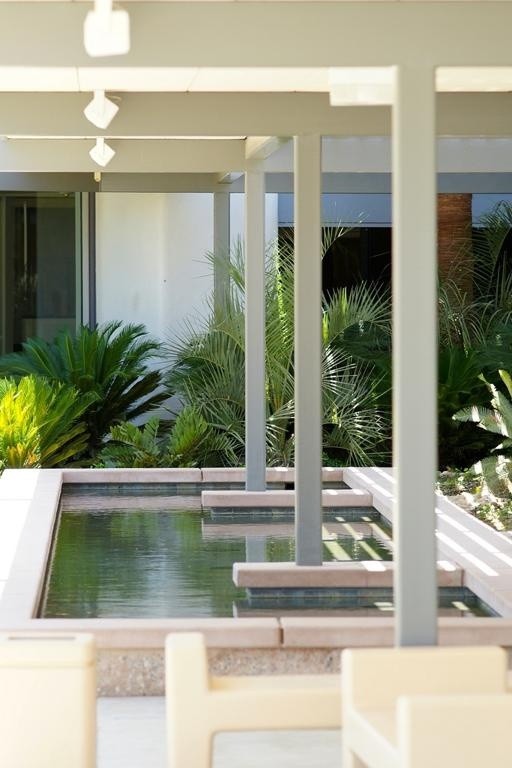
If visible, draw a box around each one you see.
[81,90,121,131]
[87,137,117,170]
[82,0,131,58]
